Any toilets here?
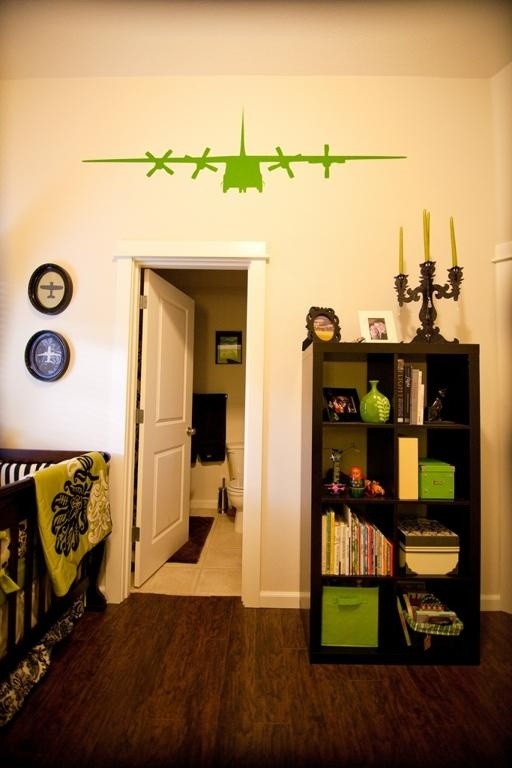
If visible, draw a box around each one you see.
[225,441,244,533]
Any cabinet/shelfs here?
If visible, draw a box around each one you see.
[299,340,481,666]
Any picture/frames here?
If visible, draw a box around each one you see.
[214,330,243,364]
[356,310,399,343]
[323,386,361,422]
[305,305,341,343]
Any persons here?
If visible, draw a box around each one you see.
[332,395,350,413]
[370,324,380,339]
[373,319,387,339]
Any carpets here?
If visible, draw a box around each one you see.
[166,516,214,564]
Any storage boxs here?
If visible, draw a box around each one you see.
[323,584,380,648]
[400,518,460,573]
[418,457,455,499]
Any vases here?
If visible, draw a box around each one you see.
[358,380,390,423]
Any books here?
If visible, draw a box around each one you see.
[397,358,425,425]
[322,502,394,576]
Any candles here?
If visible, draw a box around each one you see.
[449,215,457,266]
[398,226,404,273]
[422,208,430,261]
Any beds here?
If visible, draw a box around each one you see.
[2,448,114,729]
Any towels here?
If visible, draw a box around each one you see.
[191,392,229,467]
